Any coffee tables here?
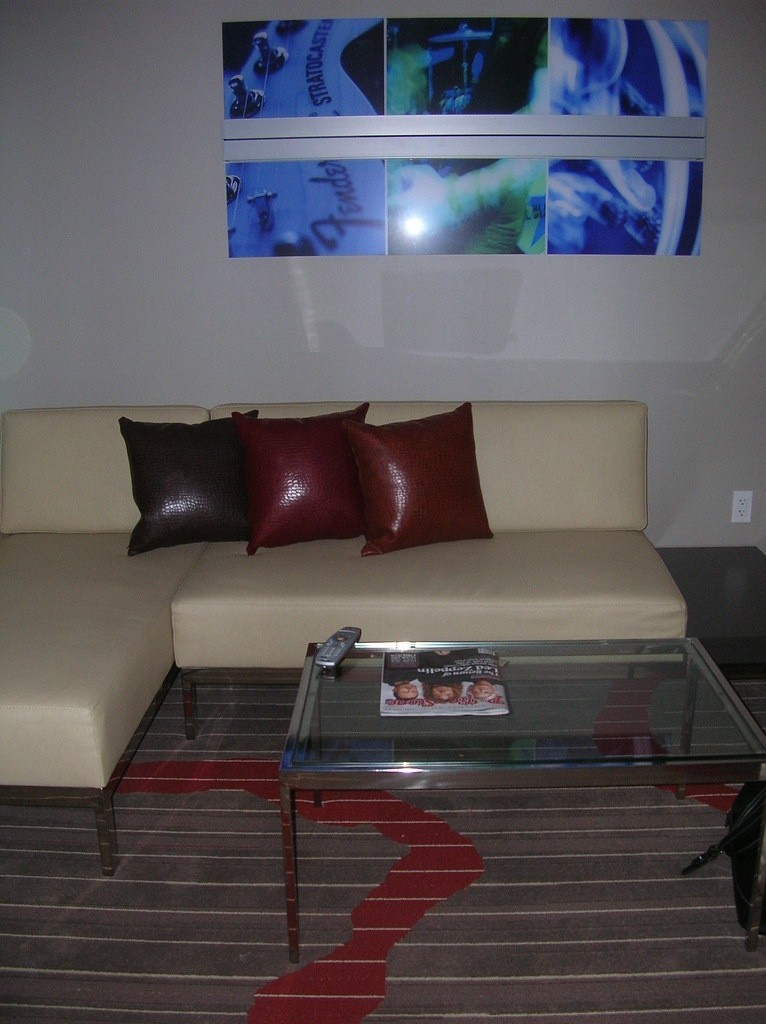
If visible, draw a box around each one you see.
[277,636,766,964]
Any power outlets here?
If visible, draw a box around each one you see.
[732,490,753,523]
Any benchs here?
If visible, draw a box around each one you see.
[0,400,699,876]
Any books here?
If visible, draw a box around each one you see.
[380,647,510,716]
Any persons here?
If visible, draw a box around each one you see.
[383,651,505,703]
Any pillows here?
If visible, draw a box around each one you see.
[341,401,495,557]
[117,409,259,558]
[230,402,370,556]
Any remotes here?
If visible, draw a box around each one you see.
[314,626,361,669]
